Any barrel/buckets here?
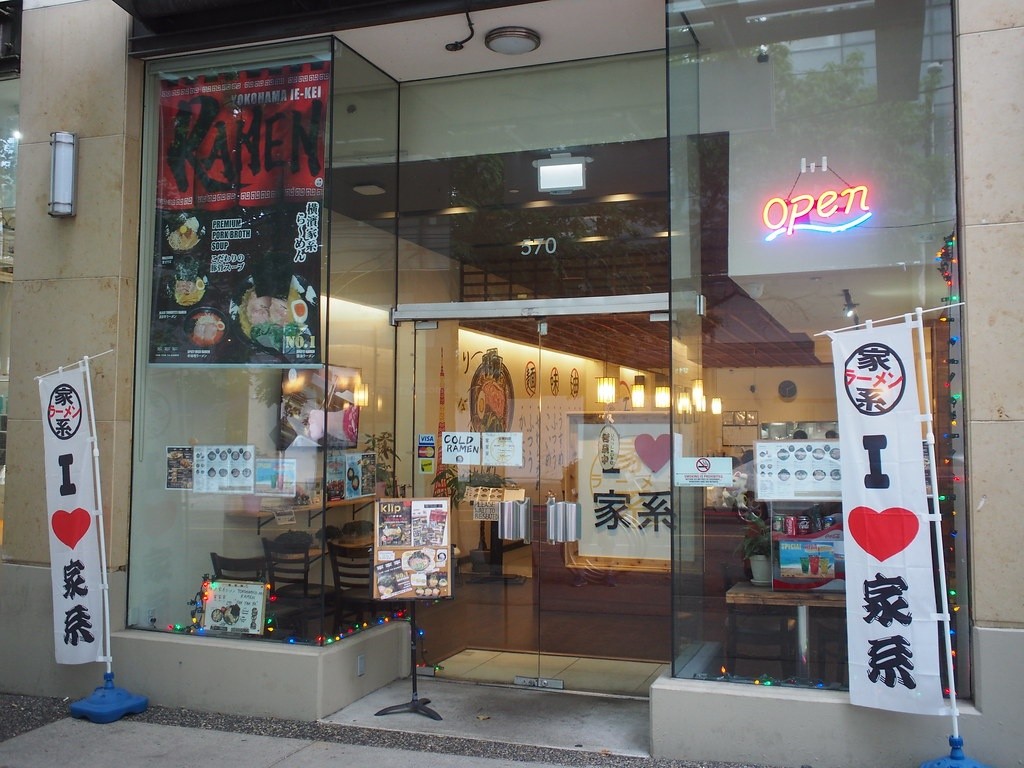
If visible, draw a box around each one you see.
[822,523,823,524]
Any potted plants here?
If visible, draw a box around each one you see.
[734,504,772,586]
[431,464,517,571]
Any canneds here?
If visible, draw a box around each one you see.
[785,516,797,535]
[772,515,784,534]
[823,516,834,530]
[811,517,822,532]
[798,516,810,535]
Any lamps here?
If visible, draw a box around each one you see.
[655,338,670,408]
[594,327,618,403]
[711,361,721,414]
[691,325,703,406]
[484,26,541,55]
[695,365,706,413]
[677,342,691,414]
[630,336,644,408]
[47,131,78,218]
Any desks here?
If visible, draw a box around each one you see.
[225,497,382,536]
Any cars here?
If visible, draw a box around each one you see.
[710,442,841,522]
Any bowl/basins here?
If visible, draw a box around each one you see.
[408,551,430,572]
[212,609,222,622]
[779,470,840,481]
[777,449,841,461]
[208,467,216,477]
[219,468,228,476]
[181,307,229,350]
[208,451,216,460]
[230,273,316,353]
[223,605,240,624]
[243,451,251,460]
[220,450,227,460]
[231,451,240,460]
[231,468,240,477]
[243,468,251,477]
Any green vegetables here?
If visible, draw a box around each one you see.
[251,322,299,346]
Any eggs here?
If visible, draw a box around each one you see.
[217,321,225,330]
[291,300,308,323]
[196,279,205,290]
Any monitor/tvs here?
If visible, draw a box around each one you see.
[537,157,585,192]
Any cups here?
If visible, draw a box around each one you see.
[819,559,829,573]
[800,557,810,574]
[808,556,820,574]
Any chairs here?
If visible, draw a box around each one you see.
[262,537,343,639]
[327,541,417,647]
[210,551,303,642]
